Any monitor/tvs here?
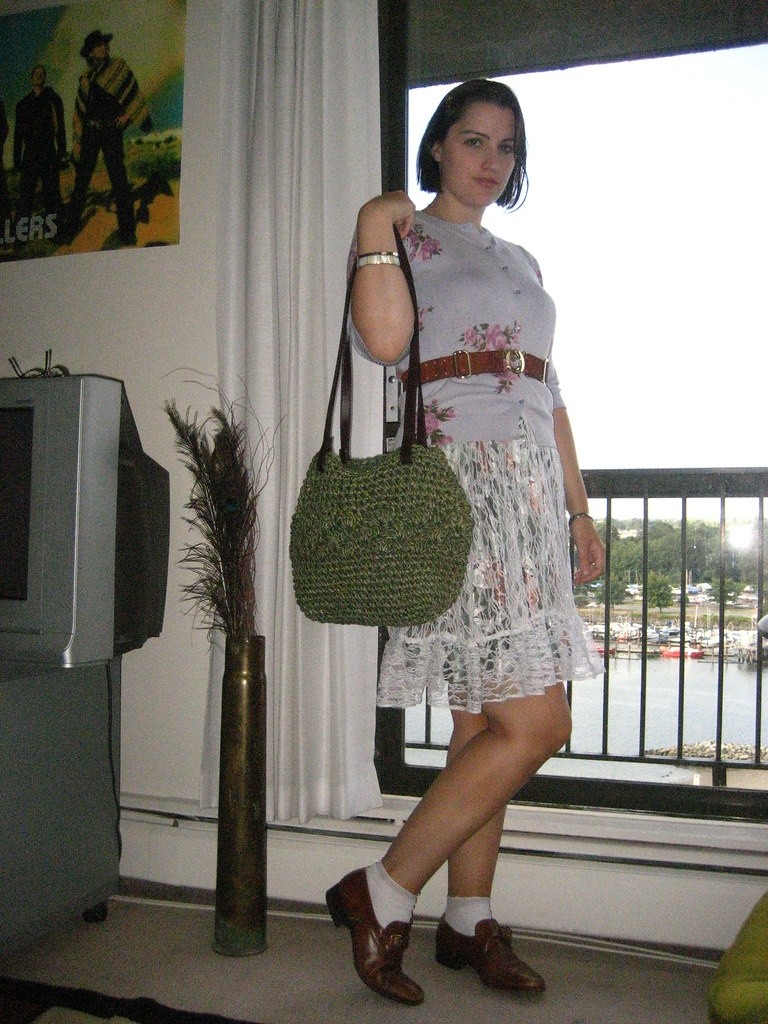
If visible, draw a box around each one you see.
[0,373,170,669]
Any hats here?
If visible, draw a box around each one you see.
[79,29,113,57]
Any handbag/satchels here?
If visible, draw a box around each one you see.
[288,444,475,627]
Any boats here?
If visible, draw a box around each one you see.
[576,607,768,666]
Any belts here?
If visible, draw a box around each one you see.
[86,119,118,129]
[401,349,550,391]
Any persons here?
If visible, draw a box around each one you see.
[325,79,604,1006]
[0,30,144,251]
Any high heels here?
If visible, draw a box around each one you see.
[326,867,424,1005]
[435,911,546,992]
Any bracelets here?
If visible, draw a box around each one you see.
[356,251,400,269]
[569,513,595,528]
